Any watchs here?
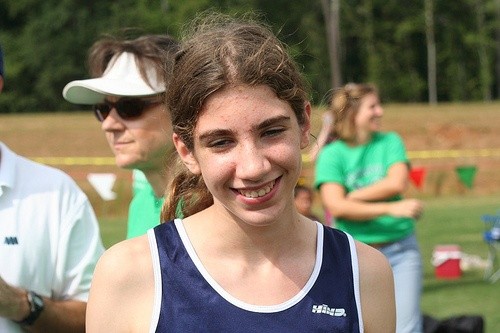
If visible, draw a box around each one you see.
[12,290,44,326]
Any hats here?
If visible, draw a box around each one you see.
[62,48,168,96]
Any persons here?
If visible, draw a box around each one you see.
[0,46,106,333]
[85,10,397,333]
[295,112,332,227]
[63,32,200,240]
[312,83,425,333]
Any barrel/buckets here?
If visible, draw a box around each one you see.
[407,167,426,189]
[435,245,461,278]
[455,167,476,187]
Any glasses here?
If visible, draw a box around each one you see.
[94,98,166,120]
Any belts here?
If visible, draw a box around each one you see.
[367,241,397,250]
